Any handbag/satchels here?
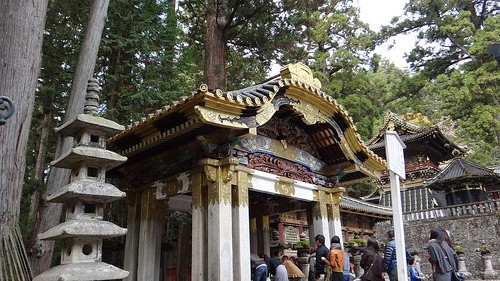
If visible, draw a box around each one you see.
[360,273,368,281]
[324,262,332,281]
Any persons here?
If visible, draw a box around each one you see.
[347,229,411,281]
[308,234,344,281]
[409,257,424,281]
[251,253,305,281]
[423,226,465,281]
